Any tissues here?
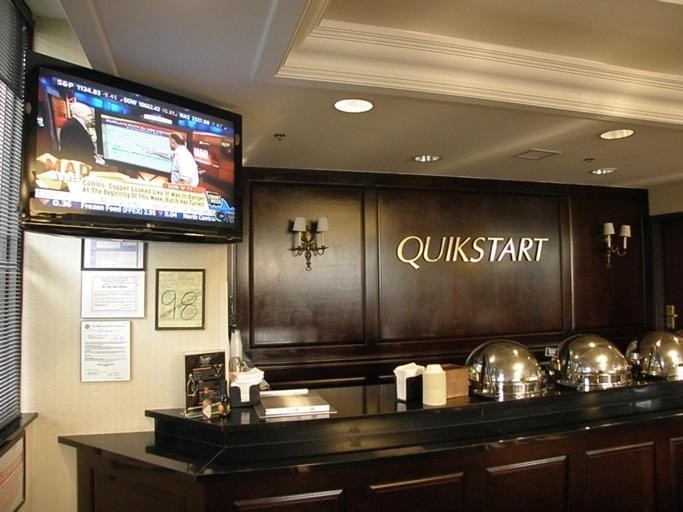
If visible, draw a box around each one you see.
[392,361,424,405]
[423,363,471,406]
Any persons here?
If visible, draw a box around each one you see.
[169,132,200,188]
[60,102,106,166]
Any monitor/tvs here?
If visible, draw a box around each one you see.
[18,50,244,243]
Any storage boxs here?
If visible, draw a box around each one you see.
[392,363,470,408]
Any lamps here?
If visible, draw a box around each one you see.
[598,222,632,271]
[289,215,331,270]
